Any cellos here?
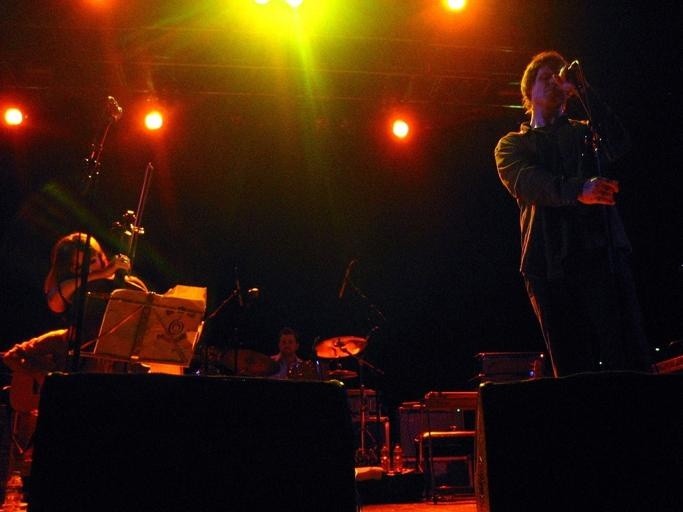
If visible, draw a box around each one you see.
[68,209,149,373]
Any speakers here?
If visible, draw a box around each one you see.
[26,365,360,512]
[479,369,683,512]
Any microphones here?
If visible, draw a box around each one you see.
[104,95,124,123]
[235,287,259,295]
[550,59,579,87]
[338,260,356,298]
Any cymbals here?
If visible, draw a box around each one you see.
[222,349,283,379]
[316,336,367,358]
[327,371,358,378]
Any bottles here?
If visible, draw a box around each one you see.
[393,442,404,474]
[380,442,390,474]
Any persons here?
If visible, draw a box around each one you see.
[263,327,306,378]
[0,229,137,511]
[490,46,657,378]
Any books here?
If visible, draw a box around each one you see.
[91,286,206,371]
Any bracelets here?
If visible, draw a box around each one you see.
[101,266,112,275]
